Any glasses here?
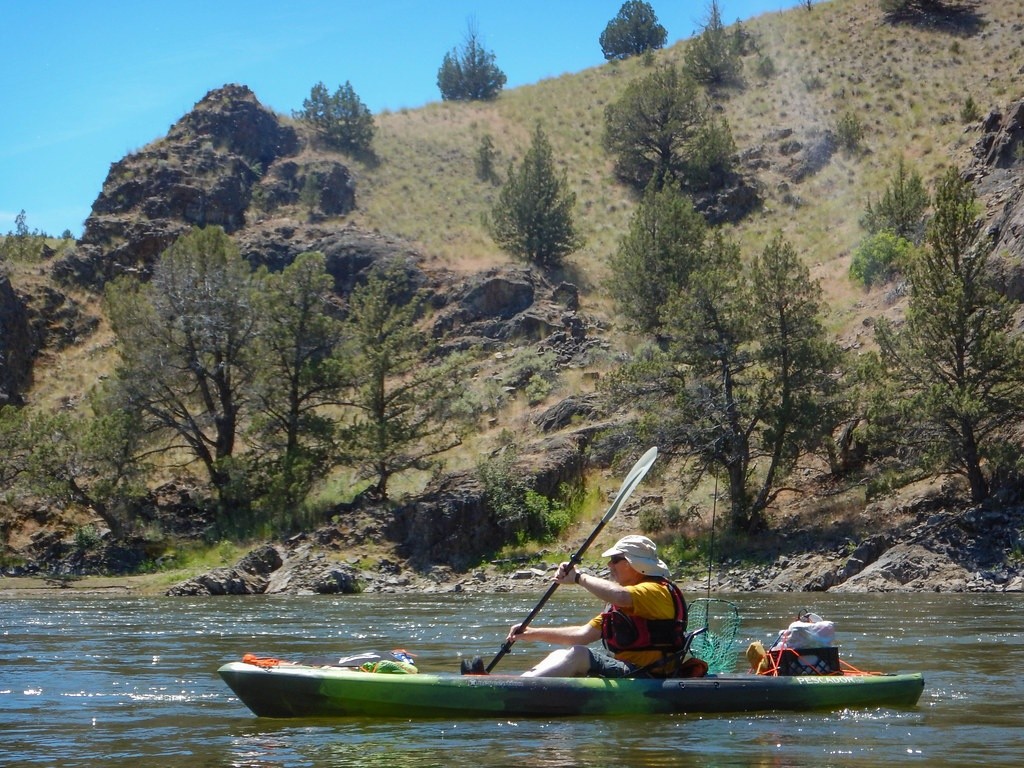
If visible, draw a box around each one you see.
[611,555,626,564]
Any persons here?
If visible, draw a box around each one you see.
[461,535,688,677]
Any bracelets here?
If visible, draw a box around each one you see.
[575,570,588,584]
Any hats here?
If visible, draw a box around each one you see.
[602,535,672,577]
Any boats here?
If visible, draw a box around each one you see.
[217,652,926,719]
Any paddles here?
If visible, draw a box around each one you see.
[485,443,660,674]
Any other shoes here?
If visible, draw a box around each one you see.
[472,655,488,675]
[461,659,473,675]
